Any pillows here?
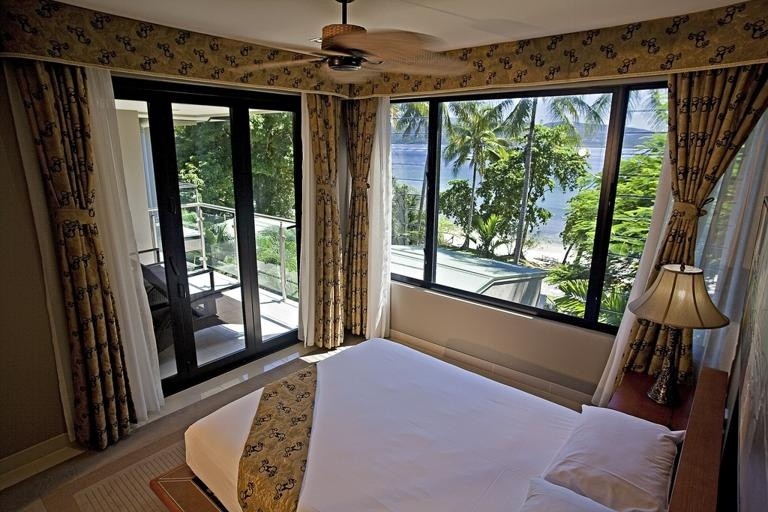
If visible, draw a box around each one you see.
[516,475,617,511]
[540,405,686,512]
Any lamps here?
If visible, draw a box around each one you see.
[627,262,729,406]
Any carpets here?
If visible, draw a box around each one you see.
[70,439,213,512]
[149,463,229,512]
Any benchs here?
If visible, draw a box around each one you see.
[127,247,223,352]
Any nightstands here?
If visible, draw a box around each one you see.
[606,367,682,429]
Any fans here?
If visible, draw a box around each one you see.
[228,0,474,86]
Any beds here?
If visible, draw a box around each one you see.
[184,338,728,511]
